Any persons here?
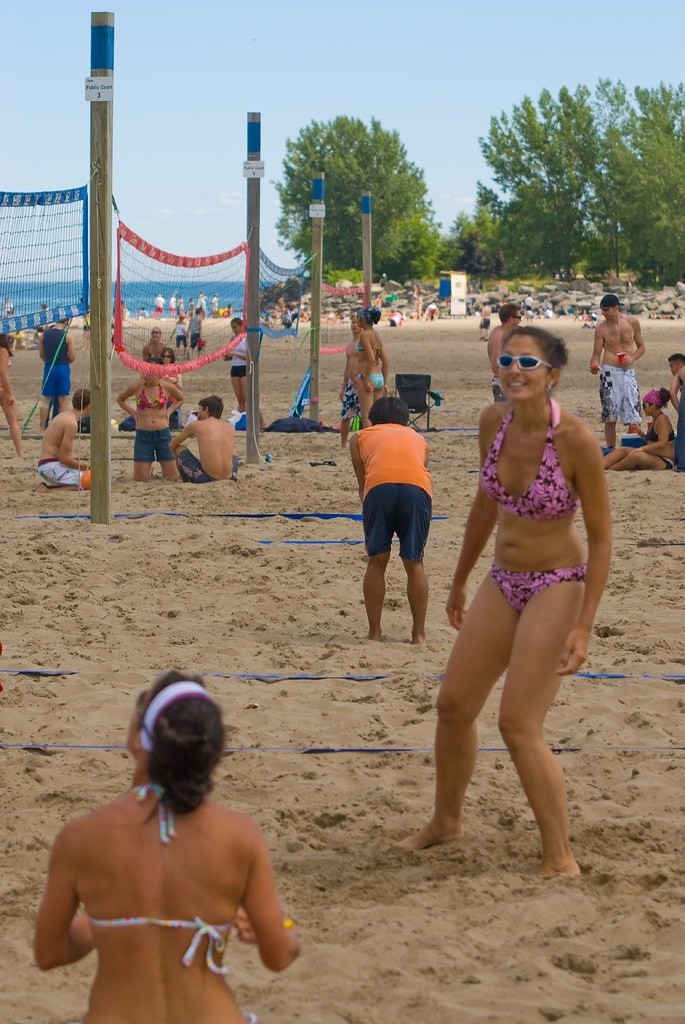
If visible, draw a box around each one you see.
[0,334,24,457]
[590,294,645,448]
[39,319,75,431]
[340,316,362,448]
[402,326,612,874]
[2,297,49,351]
[501,292,682,329]
[258,274,491,343]
[80,291,232,360]
[349,395,433,644]
[356,306,389,428]
[668,353,685,472]
[116,358,185,482]
[487,303,522,401]
[34,389,91,493]
[603,387,675,471]
[169,396,238,484]
[143,327,182,429]
[34,668,301,1023]
[227,318,266,428]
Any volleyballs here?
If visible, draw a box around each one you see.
[81,469,91,491]
[111,418,119,431]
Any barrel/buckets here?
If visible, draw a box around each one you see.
[620,434,646,448]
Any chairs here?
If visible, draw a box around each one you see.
[389,373,445,432]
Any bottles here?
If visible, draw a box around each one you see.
[265,449,271,463]
[435,392,441,406]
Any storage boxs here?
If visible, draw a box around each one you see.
[621,433,647,448]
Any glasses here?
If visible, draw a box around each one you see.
[642,402,653,408]
[512,314,522,320]
[163,355,171,358]
[152,330,161,334]
[495,353,555,372]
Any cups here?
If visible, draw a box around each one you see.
[630,422,640,434]
[617,352,626,365]
